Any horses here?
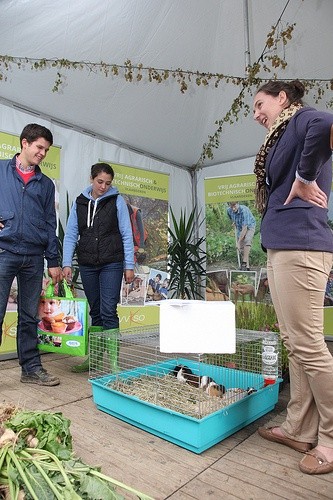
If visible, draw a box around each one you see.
[125,277,143,295]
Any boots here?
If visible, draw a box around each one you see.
[103,328,123,374]
[69,325,105,373]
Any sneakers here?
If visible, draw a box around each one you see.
[20,367,60,386]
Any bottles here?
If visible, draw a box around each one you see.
[262,340,278,385]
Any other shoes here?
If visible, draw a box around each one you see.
[258,425,312,454]
[299,446,333,475]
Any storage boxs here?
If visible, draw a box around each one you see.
[159,298,236,355]
[88,357,285,455]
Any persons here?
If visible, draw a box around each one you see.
[0,124,63,386]
[38,299,61,316]
[149,274,169,299]
[253,79,333,475]
[62,163,134,374]
[122,195,145,266]
[227,202,257,270]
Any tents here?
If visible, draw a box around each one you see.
[0,0,333,358]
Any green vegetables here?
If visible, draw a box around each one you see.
[0,409,156,500]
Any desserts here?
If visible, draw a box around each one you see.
[42,313,76,333]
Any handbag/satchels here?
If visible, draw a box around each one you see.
[36,278,88,357]
